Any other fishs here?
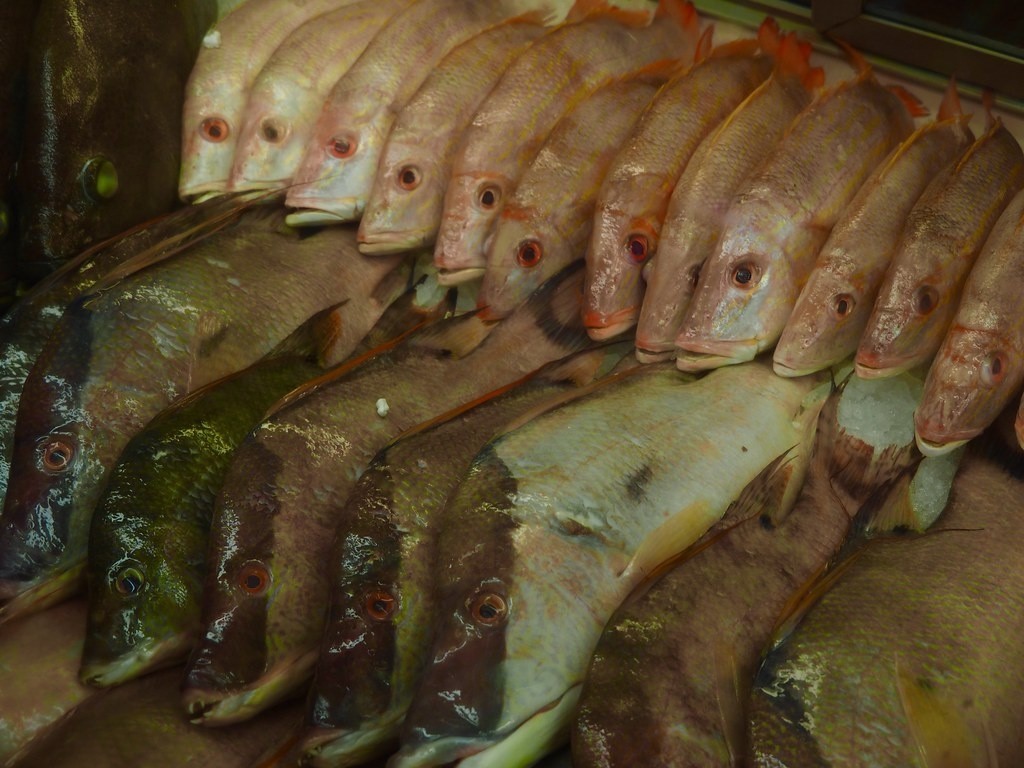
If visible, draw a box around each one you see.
[0,0,1024,768]
